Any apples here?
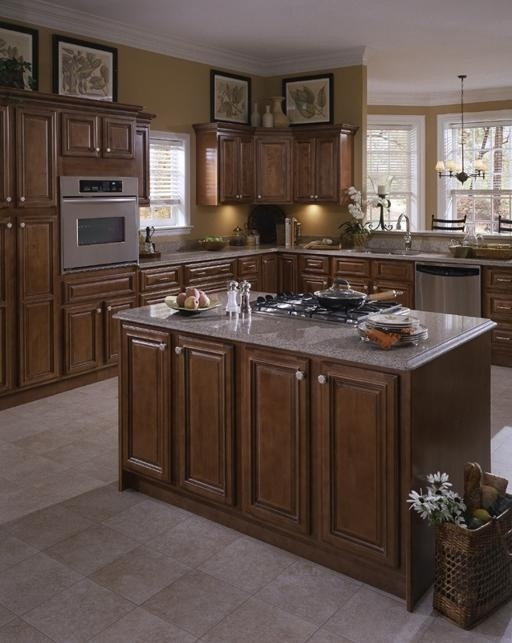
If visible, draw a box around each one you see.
[205,236,224,241]
[176,287,210,309]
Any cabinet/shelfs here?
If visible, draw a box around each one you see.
[191,121,361,209]
[139,240,279,306]
[134,110,158,209]
[279,245,512,371]
[2,88,145,407]
[109,286,500,612]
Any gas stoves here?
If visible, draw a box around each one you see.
[241,291,410,326]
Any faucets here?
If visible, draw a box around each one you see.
[396,214,413,250]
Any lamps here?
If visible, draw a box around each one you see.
[434,74,487,185]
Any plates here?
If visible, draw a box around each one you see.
[357,315,432,346]
[164,295,221,313]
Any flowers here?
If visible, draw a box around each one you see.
[403,469,469,531]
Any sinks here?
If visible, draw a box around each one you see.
[349,248,420,256]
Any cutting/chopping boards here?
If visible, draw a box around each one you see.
[306,241,342,250]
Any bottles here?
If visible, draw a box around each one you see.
[238,281,251,320]
[262,104,274,128]
[230,226,244,246]
[144,226,155,253]
[224,280,240,319]
[247,228,260,246]
[284,216,301,246]
[251,99,260,127]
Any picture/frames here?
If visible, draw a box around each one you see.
[0,22,39,90]
[52,35,118,104]
[282,73,334,127]
[210,70,251,125]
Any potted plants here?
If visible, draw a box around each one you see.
[0,59,32,90]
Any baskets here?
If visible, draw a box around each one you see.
[431,493,511,631]
[198,239,229,251]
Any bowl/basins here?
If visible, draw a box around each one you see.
[448,245,470,258]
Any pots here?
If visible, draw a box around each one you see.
[312,279,404,314]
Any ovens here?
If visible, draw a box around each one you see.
[57,174,140,278]
